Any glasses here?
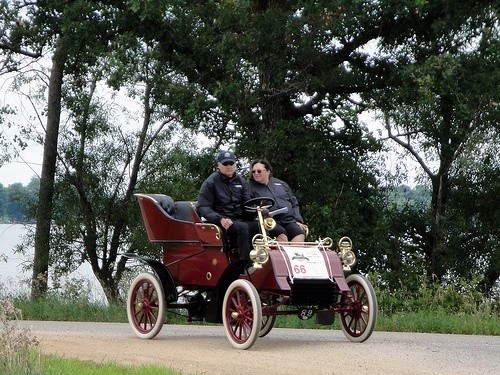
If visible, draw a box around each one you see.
[252,169,261,173]
[222,162,234,166]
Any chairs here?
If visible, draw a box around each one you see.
[175,198,194,222]
[149,192,175,222]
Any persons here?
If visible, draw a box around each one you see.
[248,158,309,245]
[195,151,269,263]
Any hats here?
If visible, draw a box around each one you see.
[217,151,236,163]
